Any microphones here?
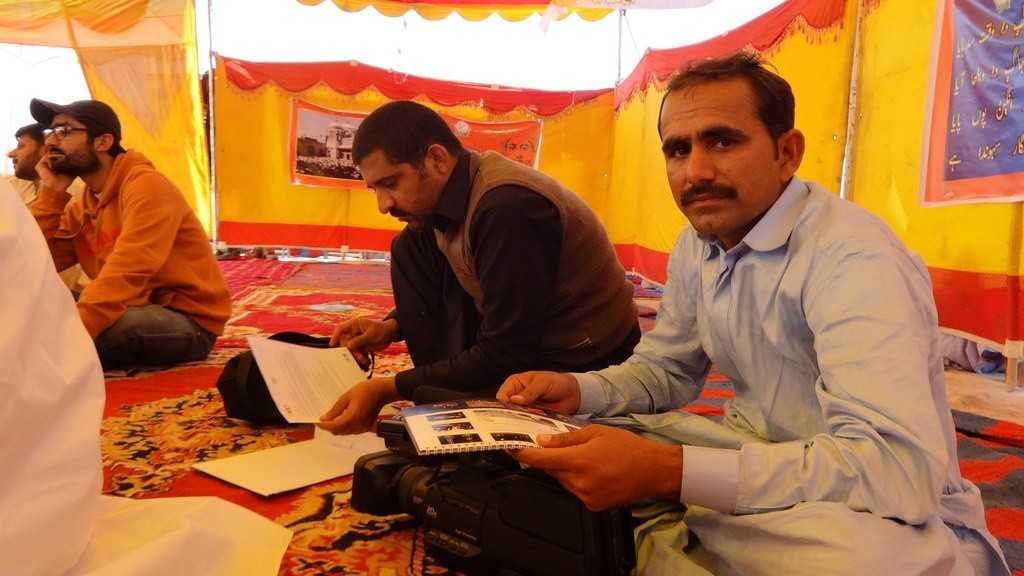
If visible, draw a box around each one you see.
[412,387,474,406]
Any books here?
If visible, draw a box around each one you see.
[401,397,583,455]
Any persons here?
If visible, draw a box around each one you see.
[313,103,643,435]
[7,99,232,370]
[495,52,966,576]
[0,177,105,576]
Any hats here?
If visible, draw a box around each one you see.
[31,98,121,140]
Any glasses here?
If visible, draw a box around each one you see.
[42,126,89,140]
